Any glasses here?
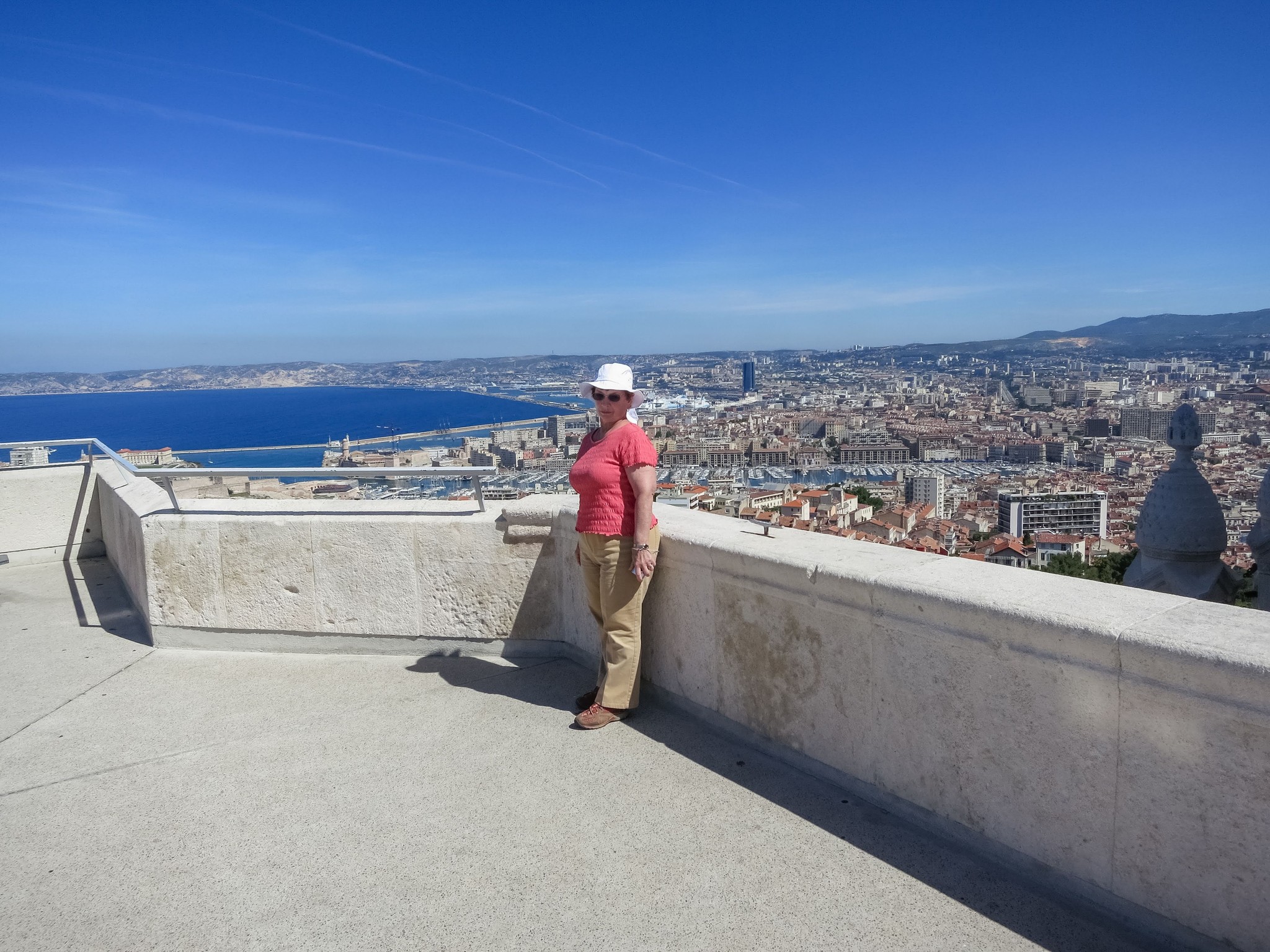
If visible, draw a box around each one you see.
[592,393,628,402]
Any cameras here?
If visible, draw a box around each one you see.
[631,566,644,577]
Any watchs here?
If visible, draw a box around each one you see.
[633,543,649,549]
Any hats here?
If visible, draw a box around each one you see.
[579,363,644,409]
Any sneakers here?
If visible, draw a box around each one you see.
[575,686,599,708]
[575,702,629,729]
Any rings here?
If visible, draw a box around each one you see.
[648,565,653,569]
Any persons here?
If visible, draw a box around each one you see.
[569,363,660,729]
